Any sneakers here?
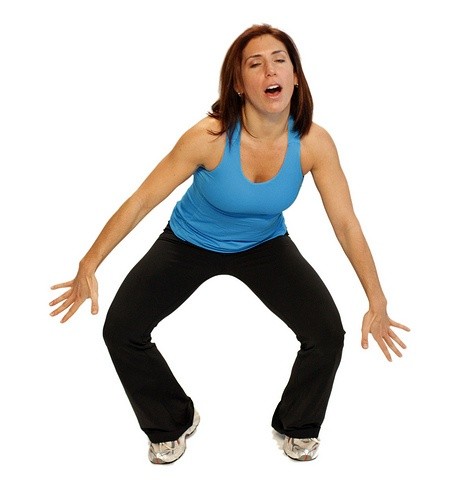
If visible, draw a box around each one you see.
[149,409,200,463]
[282,434,318,460]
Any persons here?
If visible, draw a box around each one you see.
[47,25,410,464]
